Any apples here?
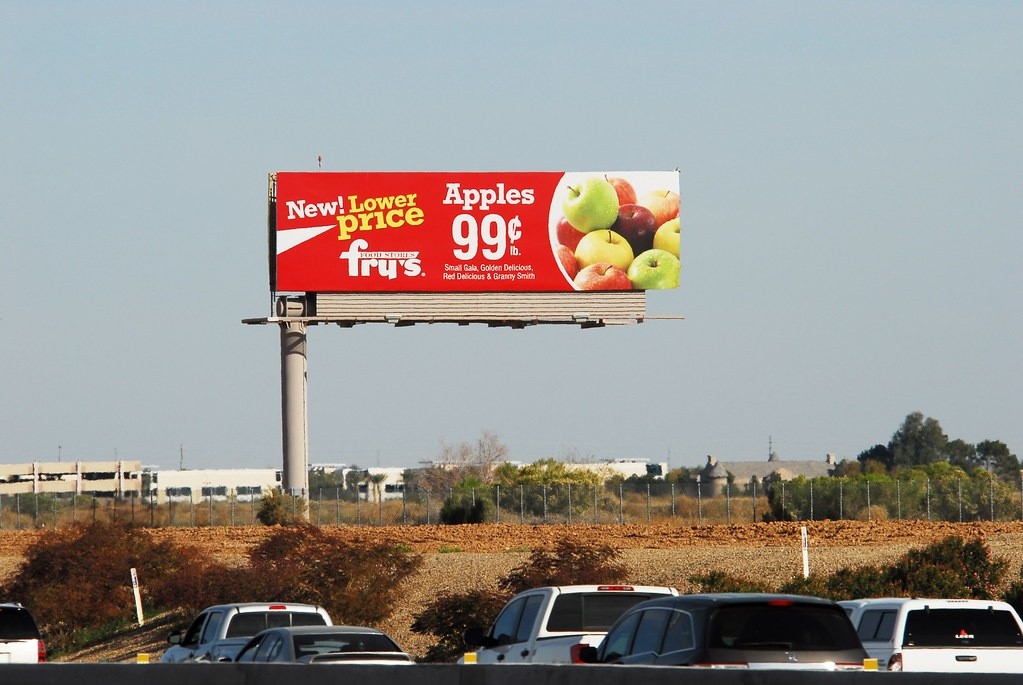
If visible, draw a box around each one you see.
[553,173,680,291]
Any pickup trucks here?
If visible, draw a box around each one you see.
[159,603,361,663]
[835,599,1023,674]
[457,584,680,666]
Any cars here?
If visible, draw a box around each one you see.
[217,626,417,665]
[578,594,870,669]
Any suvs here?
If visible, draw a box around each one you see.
[0,602,46,664]
